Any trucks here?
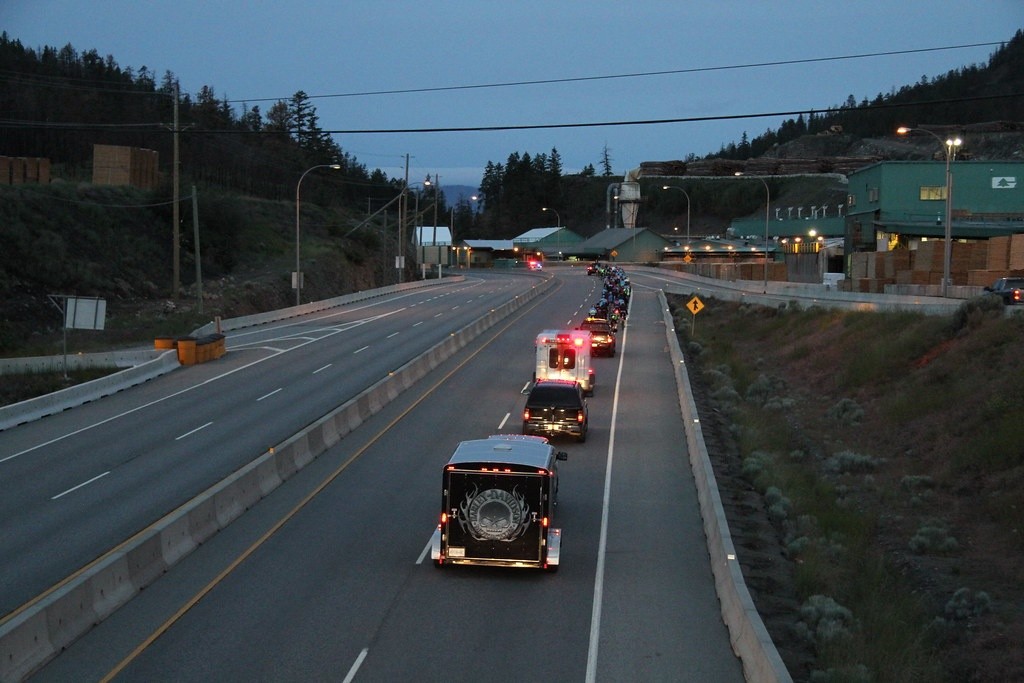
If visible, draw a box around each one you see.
[432,435,569,573]
[533,329,595,397]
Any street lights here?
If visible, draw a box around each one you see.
[899,126,951,297]
[614,196,635,261]
[450,196,477,265]
[734,171,769,286]
[398,182,431,283]
[542,208,560,261]
[663,186,690,264]
[296,165,340,305]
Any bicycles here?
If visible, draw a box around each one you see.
[588,265,631,331]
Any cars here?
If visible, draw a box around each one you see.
[528,262,543,271]
[523,378,588,441]
[574,318,618,357]
[984,277,1024,305]
[706,235,720,241]
[587,263,601,275]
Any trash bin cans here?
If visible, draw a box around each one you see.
[155,333,226,366]
